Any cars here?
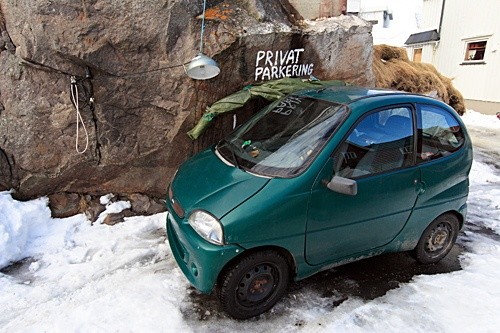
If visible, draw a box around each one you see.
[166,84,474,321]
